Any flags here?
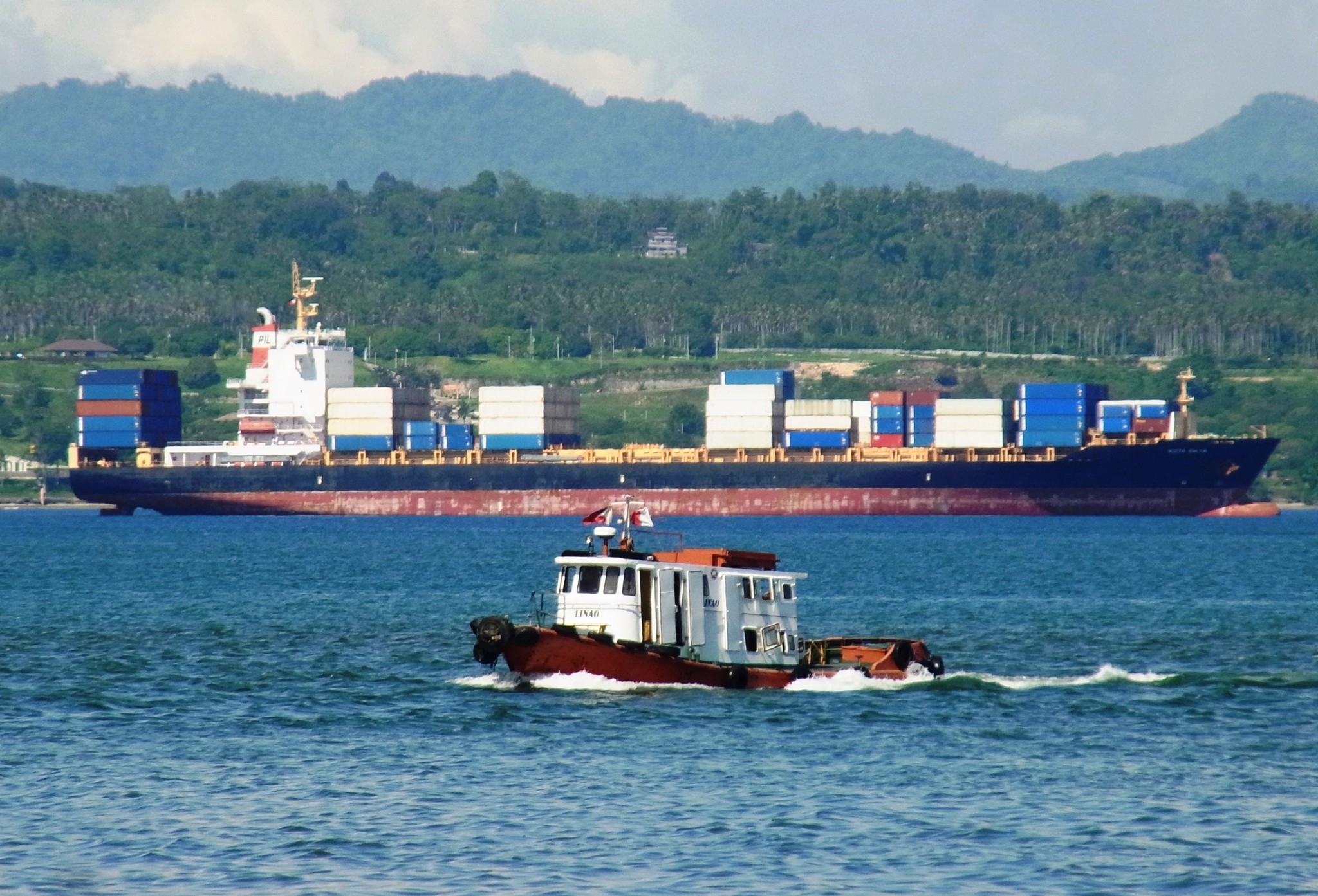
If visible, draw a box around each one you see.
[289,298,296,306]
[618,506,653,527]
[582,507,612,526]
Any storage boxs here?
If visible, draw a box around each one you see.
[73,368,183,461]
[703,367,1178,450]
[326,384,582,452]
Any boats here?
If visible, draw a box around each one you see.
[471,493,943,693]
[65,253,1294,519]
